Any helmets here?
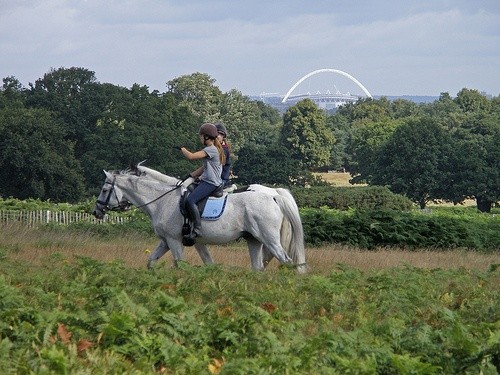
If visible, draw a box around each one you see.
[200,124,218,138]
[215,124,227,137]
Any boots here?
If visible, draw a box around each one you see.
[183,205,203,239]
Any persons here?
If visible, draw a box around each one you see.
[182,123,231,188]
[172,123,226,239]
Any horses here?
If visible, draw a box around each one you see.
[114,160,298,271]
[92,169,306,275]
